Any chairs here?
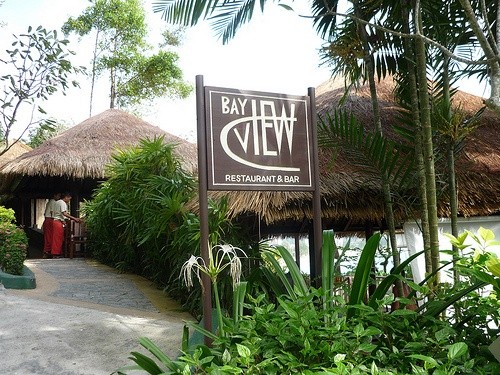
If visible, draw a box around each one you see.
[65,218,87,259]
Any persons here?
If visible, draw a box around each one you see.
[50,190,83,260]
[42,190,61,259]
